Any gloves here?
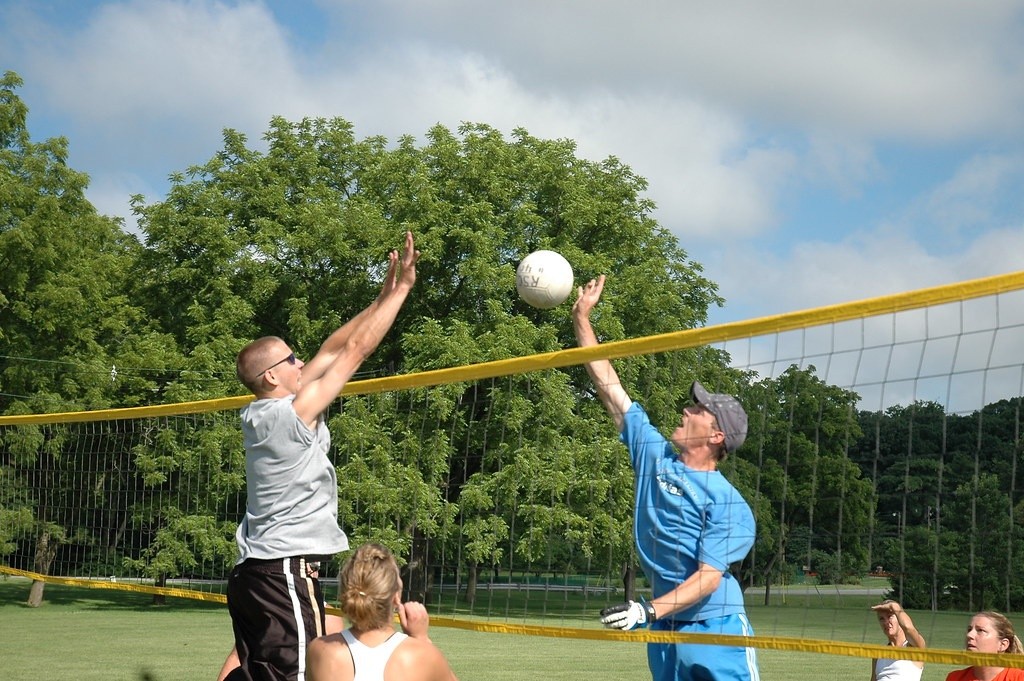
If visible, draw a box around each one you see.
[600,600,656,631]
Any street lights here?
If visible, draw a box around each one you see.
[893,513,901,534]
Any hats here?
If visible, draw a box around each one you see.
[689,380,749,453]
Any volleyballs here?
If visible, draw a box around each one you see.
[515,249,575,311]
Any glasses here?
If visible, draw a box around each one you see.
[255,354,295,378]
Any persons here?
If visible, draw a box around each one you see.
[871,600,925,681]
[305,544,459,681]
[946,612,1024,681]
[217,562,343,681]
[572,276,760,681]
[226,231,420,681]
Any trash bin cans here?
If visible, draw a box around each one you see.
[110,576,116,583]
